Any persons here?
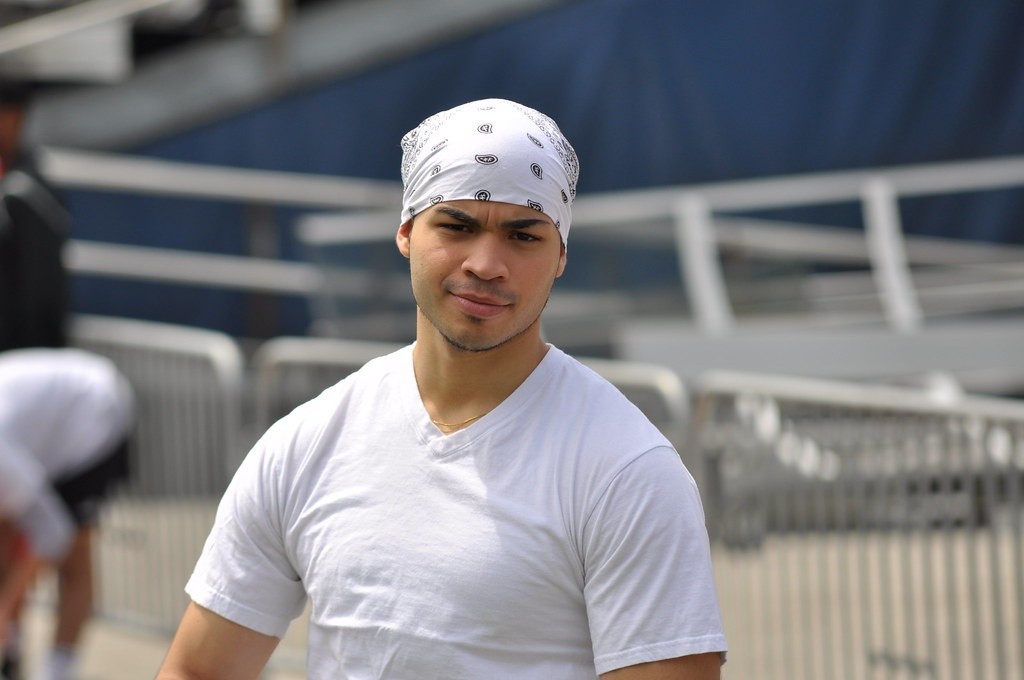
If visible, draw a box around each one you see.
[0,347,139,679]
[152,97,729,680]
[0,60,74,344]
